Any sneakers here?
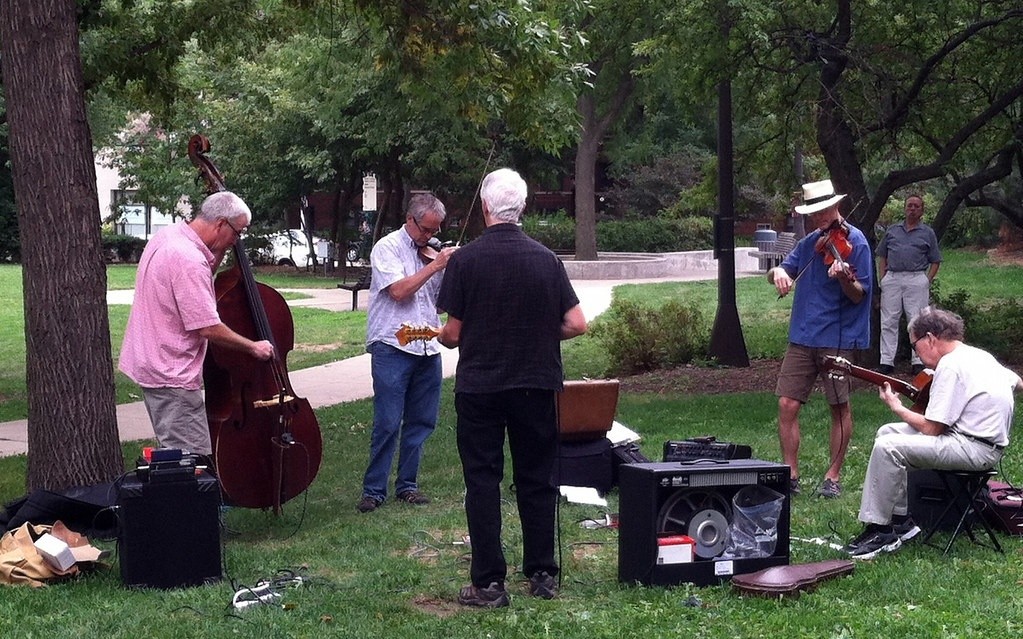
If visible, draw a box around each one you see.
[458,580,510,607]
[893,514,921,544]
[841,528,902,559]
[530,572,555,599]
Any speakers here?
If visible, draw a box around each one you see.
[112,474,225,589]
[899,465,992,533]
[617,460,795,587]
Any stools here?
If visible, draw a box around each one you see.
[923,469,1004,557]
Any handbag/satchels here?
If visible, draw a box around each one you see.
[0,520,111,589]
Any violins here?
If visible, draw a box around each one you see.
[814,223,858,284]
[417,237,452,261]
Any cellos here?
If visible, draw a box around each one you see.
[183,135,323,521]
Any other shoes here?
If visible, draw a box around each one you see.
[399,491,428,503]
[876,365,893,375]
[790,474,802,494]
[913,364,923,374]
[818,478,841,499]
[359,497,378,512]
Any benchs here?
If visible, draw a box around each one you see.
[748,232,799,274]
[337,266,372,312]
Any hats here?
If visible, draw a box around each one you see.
[795,180,847,214]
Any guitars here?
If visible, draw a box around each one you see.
[824,351,936,417]
[394,320,442,347]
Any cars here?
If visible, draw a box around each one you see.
[223,228,330,268]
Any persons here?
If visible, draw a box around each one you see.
[841,304,1023,559]
[875,194,942,376]
[118,190,274,457]
[767,179,872,498]
[436,169,586,608]
[358,193,460,514]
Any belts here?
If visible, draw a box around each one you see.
[964,434,1004,450]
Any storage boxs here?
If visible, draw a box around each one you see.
[34,533,111,572]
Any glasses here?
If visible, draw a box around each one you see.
[225,218,241,241]
[413,216,441,235]
[909,335,926,351]
